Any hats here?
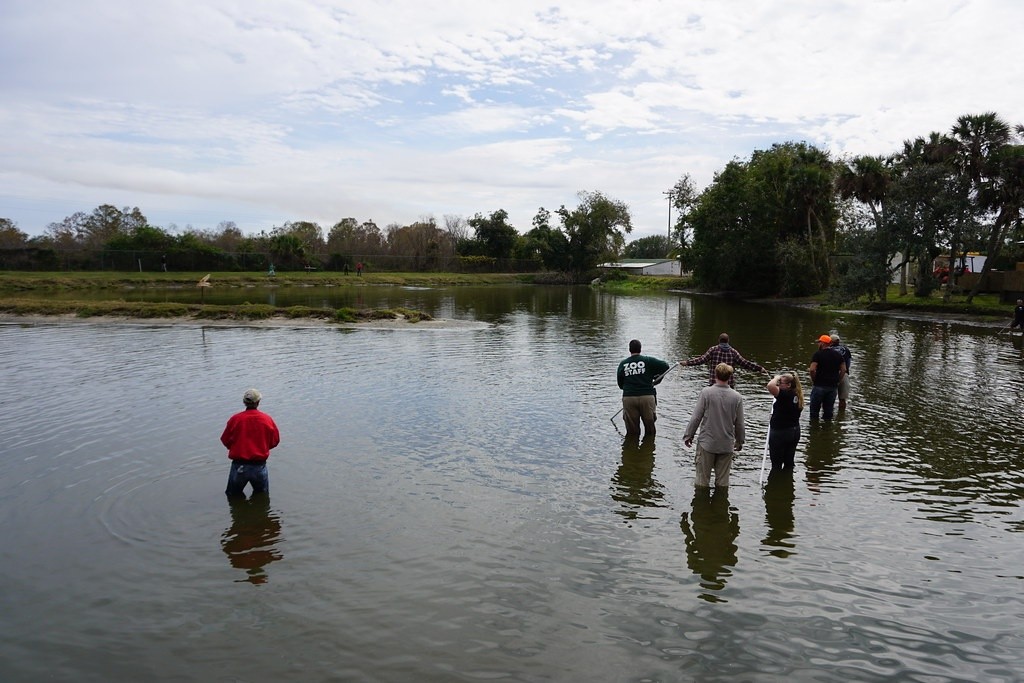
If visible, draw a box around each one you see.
[830,334,840,342]
[243,389,262,403]
[815,335,832,343]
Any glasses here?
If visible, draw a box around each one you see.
[780,382,788,384]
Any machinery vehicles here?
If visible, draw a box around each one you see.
[934,255,975,284]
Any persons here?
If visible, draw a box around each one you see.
[343,262,349,275]
[680,333,767,390]
[219,390,280,495]
[809,334,846,421]
[1010,300,1024,333]
[679,488,740,603]
[682,363,746,487]
[610,437,664,528]
[803,424,834,490]
[762,470,798,559]
[222,493,284,585]
[267,263,275,277]
[617,340,669,436]
[161,255,168,272]
[767,370,804,470]
[305,263,310,276]
[356,262,362,276]
[829,334,851,408]
